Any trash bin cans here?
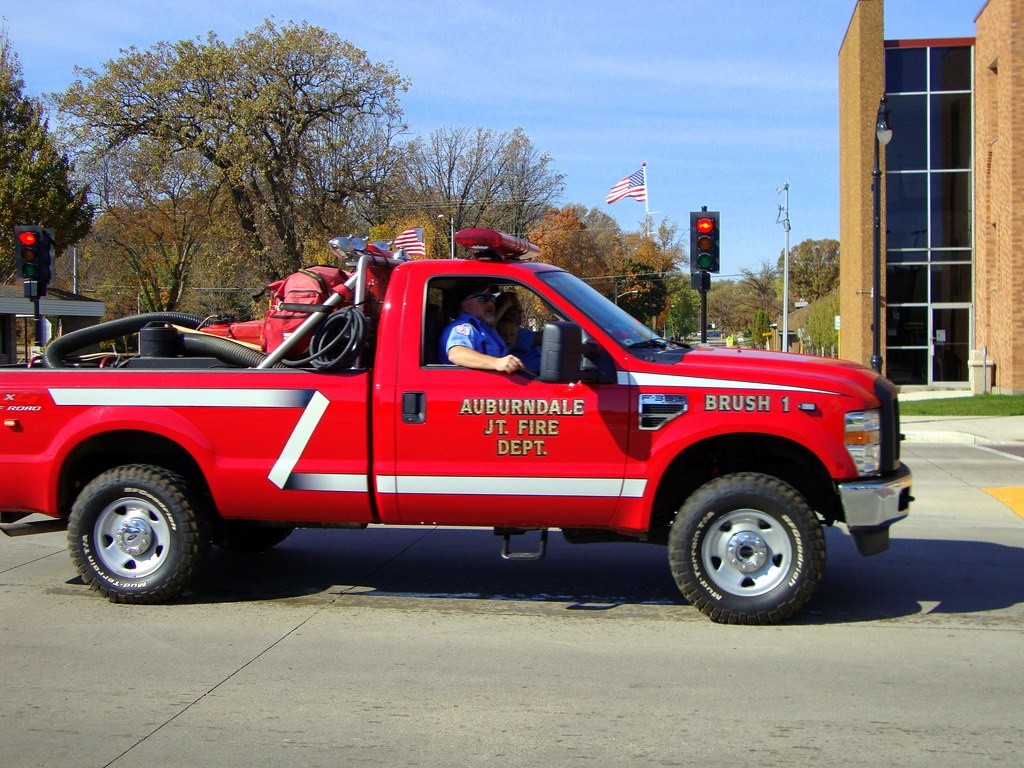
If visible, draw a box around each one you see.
[968,351,993,395]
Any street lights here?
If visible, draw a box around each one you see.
[438,214,454,260]
[869,97,894,377]
[615,283,639,306]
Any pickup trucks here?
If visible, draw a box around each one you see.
[0,227,915,627]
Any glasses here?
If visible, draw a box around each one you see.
[465,294,496,303]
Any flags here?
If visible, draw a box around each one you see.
[606,167,645,205]
[395,226,426,258]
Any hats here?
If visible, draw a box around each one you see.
[460,279,500,302]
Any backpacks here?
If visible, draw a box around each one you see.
[259,265,358,357]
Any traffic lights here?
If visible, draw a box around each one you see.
[14,223,55,284]
[689,211,720,273]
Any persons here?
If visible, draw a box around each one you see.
[438,280,542,374]
[673,330,682,342]
